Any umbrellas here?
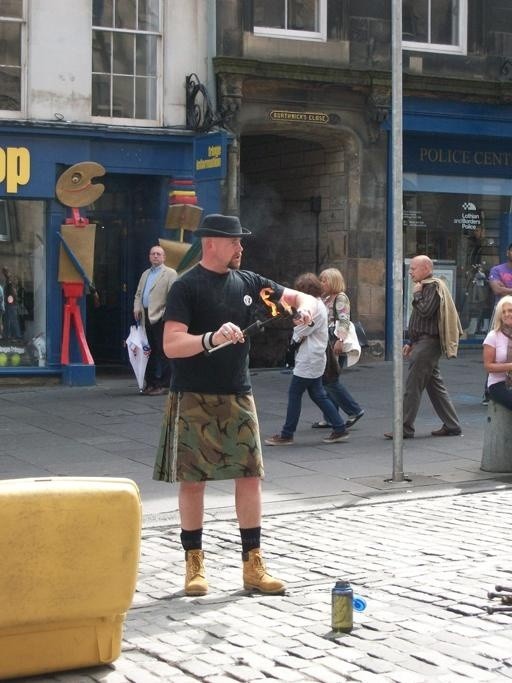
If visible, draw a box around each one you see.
[126,319,152,393]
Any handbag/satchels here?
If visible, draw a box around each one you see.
[322,341,340,384]
[328,322,368,346]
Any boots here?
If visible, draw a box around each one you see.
[242,547,286,594]
[186,549,208,596]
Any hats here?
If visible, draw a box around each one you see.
[195,214,252,237]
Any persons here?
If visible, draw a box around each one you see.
[264,272,350,446]
[153,214,318,597]
[481,295,512,410]
[133,245,178,395]
[383,254,462,439]
[488,243,512,331]
[312,268,365,429]
[463,226,500,336]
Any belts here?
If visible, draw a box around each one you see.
[411,334,439,342]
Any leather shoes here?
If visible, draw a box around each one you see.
[311,421,333,427]
[143,386,170,396]
[344,413,364,428]
[432,425,461,435]
[385,429,414,438]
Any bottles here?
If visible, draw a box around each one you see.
[332,579,353,633]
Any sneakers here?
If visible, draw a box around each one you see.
[323,431,349,442]
[265,436,294,446]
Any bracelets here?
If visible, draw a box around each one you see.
[202,331,215,352]
